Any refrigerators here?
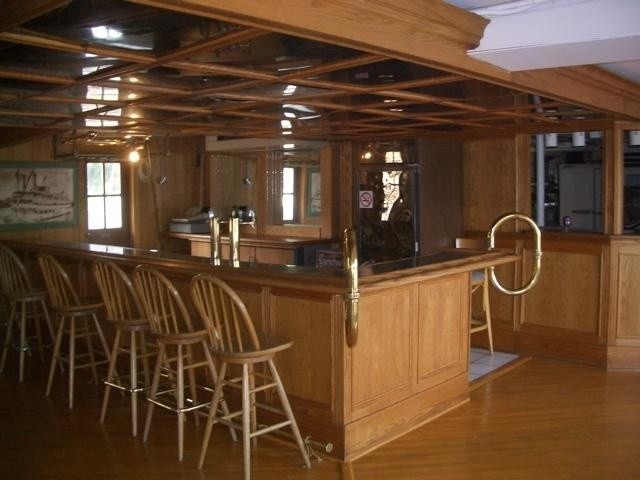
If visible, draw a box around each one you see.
[556,162,640,237]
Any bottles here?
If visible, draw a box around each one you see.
[232,204,255,222]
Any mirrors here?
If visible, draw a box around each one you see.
[209,151,322,226]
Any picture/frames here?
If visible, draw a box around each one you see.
[0,160,79,231]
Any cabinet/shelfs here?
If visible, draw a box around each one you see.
[190,239,328,266]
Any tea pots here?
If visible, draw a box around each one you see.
[558,215,577,232]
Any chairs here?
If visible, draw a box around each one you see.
[0,244,65,382]
[187,273,312,479]
[91,261,187,438]
[35,252,126,410]
[455,238,494,357]
[131,264,238,461]
